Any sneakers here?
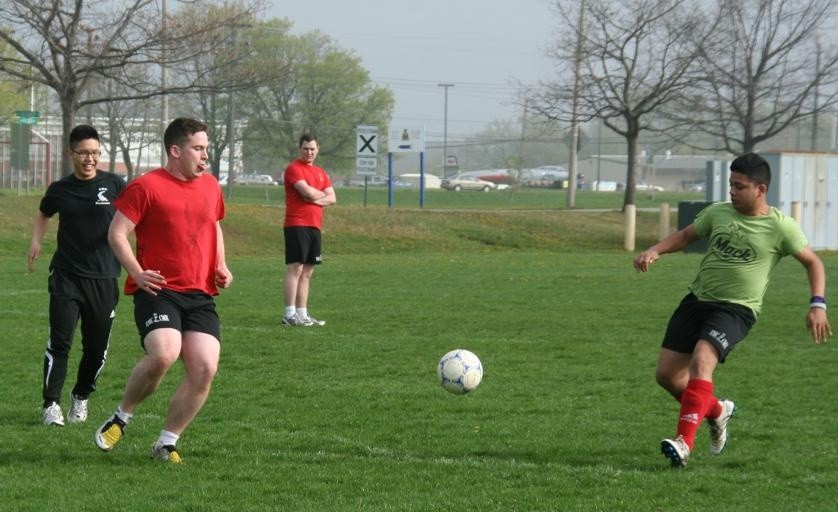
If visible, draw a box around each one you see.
[660,434,691,468]
[709,400,736,455]
[41,400,65,426]
[281,313,314,327]
[66,392,88,426]
[301,314,325,326]
[94,413,128,452]
[149,443,184,465]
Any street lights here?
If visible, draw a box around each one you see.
[437,83,456,178]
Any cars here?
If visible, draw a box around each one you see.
[539,166,569,182]
[356,175,415,188]
[219,174,284,187]
[442,174,497,193]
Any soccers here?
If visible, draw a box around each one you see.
[438,350,484,394]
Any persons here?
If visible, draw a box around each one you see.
[630,152,834,466]
[25,123,131,428]
[90,117,237,465]
[281,133,339,329]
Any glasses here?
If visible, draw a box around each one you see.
[73,150,101,158]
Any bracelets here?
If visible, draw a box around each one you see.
[809,296,827,310]
